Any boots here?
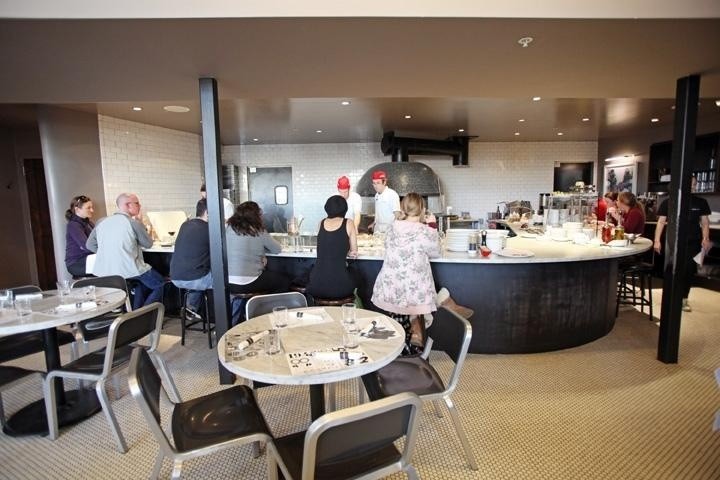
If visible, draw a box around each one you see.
[434,286,474,320]
[409,314,424,348]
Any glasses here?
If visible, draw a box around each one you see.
[371,181,384,185]
[338,190,349,194]
[77,195,86,204]
[124,199,139,206]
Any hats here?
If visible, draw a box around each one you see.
[371,171,385,179]
[324,194,348,216]
[336,176,350,189]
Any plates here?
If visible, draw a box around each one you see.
[482,229,510,250]
[551,227,565,242]
[562,222,584,241]
[496,249,535,258]
[445,229,480,252]
[583,228,595,243]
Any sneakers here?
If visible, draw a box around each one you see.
[681,304,691,312]
[184,308,205,323]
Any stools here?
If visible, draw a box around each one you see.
[616,261,653,321]
[180,288,216,349]
[161,277,181,329]
[289,286,306,293]
[125,279,137,296]
[617,270,645,305]
[230,293,260,327]
[391,314,429,364]
[314,297,354,305]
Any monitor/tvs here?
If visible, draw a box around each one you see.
[554,166,594,191]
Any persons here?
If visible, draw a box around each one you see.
[653,175,711,312]
[603,192,621,227]
[64,196,99,277]
[371,191,474,346]
[608,192,646,273]
[85,193,164,311]
[305,195,367,300]
[597,189,607,219]
[224,201,283,294]
[367,170,401,234]
[333,176,363,235]
[200,183,233,222]
[169,198,213,322]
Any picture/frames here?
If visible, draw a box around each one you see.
[603,163,637,197]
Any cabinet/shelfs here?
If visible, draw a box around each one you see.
[645,132,720,292]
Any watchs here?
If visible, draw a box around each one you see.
[701,237,710,241]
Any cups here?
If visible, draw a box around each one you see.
[343,327,360,349]
[82,286,95,301]
[167,227,176,245]
[56,280,70,296]
[294,238,304,252]
[342,303,357,324]
[265,331,280,356]
[14,296,31,317]
[280,236,288,250]
[1,289,13,311]
[272,306,288,329]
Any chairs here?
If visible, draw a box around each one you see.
[72,275,137,400]
[0,285,84,428]
[359,306,478,471]
[267,392,421,480]
[46,302,182,454]
[246,292,336,459]
[128,347,274,480]
